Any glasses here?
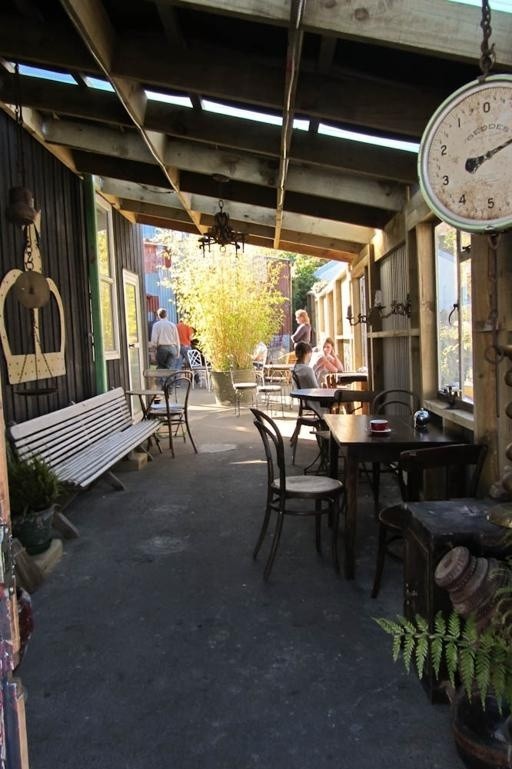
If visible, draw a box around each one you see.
[196,174,249,259]
[345,288,413,330]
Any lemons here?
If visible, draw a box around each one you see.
[413,407,431,426]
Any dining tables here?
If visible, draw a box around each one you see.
[368,509,511,769]
[4,443,60,555]
[144,223,290,409]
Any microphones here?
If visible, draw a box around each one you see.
[256,386,284,421]
[233,383,257,418]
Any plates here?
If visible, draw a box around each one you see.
[401,498,512,700]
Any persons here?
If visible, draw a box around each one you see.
[312,336,346,388]
[148,307,181,395]
[289,341,321,399]
[250,340,269,371]
[173,311,198,388]
[289,307,314,351]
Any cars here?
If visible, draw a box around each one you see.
[368,418,388,431]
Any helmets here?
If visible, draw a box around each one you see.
[365,427,392,433]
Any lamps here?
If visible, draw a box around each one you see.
[203,350,216,391]
[147,371,199,457]
[369,445,489,600]
[251,349,265,385]
[248,407,342,585]
[186,349,209,390]
[289,368,419,522]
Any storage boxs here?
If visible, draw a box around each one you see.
[8,386,168,540]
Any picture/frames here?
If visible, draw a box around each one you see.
[125,390,165,456]
[266,364,293,388]
[321,413,473,580]
